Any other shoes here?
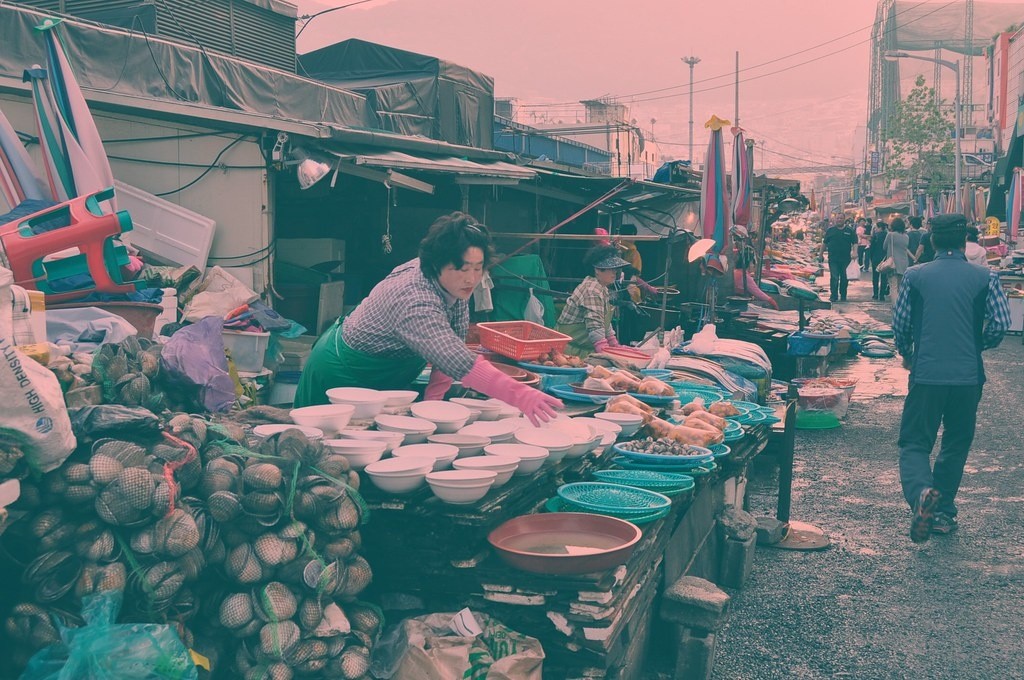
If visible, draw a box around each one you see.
[864,267,869,272]
[865,245,870,250]
[860,266,863,270]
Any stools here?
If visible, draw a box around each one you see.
[0,186,148,302]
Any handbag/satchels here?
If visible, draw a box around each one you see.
[846,257,862,281]
[875,257,896,274]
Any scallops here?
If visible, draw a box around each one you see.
[2,336,385,680]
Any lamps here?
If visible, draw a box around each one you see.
[674,228,716,262]
[280,156,333,190]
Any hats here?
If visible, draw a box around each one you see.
[929,213,967,231]
[586,243,631,269]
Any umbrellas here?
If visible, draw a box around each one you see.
[811,189,816,211]
[961,182,987,224]
[0,20,120,257]
[820,197,824,219]
[1006,167,1024,242]
[910,190,957,218]
[731,127,751,297]
[700,115,731,324]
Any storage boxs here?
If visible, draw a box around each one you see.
[787,331,832,356]
[221,331,271,373]
[154,288,178,333]
[476,320,573,361]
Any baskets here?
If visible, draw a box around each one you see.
[476,316,783,525]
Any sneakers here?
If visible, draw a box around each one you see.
[910,486,941,543]
[931,511,958,534]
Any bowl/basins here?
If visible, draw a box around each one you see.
[728,295,750,304]
[739,312,761,318]
[487,512,642,572]
[253,346,673,505]
[47,302,164,341]
[649,287,680,302]
[794,389,844,428]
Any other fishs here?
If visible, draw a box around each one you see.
[623,279,897,398]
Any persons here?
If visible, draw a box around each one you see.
[893,213,1013,545]
[620,224,658,293]
[907,217,922,266]
[735,251,779,309]
[883,218,909,310]
[295,211,565,427]
[914,217,932,264]
[965,227,988,267]
[870,223,888,300]
[820,213,856,302]
[844,217,882,272]
[556,244,631,358]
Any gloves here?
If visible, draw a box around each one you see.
[424,368,454,400]
[768,297,779,311]
[607,336,620,348]
[462,354,565,429]
[593,340,609,354]
